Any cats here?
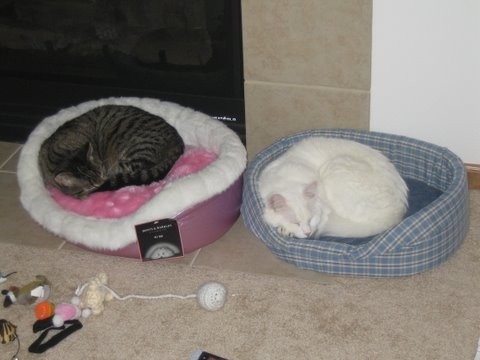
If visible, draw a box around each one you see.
[39,104,185,199]
[258,136,410,240]
